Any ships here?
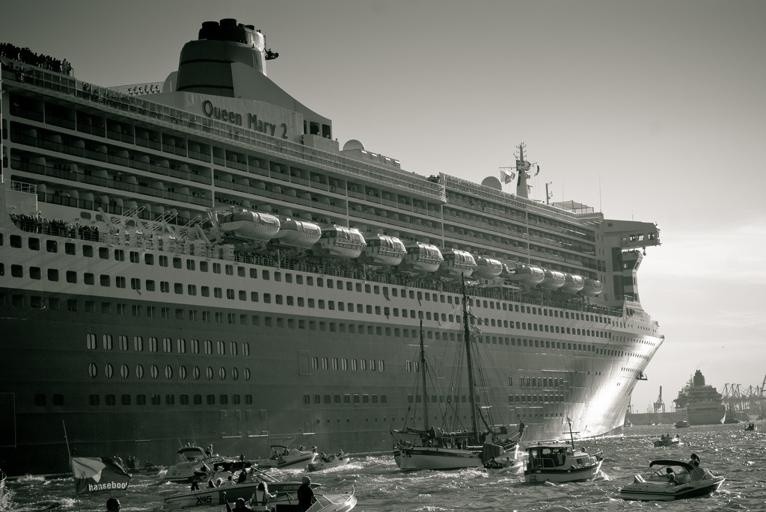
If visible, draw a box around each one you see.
[0,16,670,475]
[674,369,727,426]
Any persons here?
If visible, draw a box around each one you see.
[11,210,99,242]
[519,419,525,439]
[689,454,700,466]
[430,425,435,443]
[537,441,570,455]
[660,432,681,440]
[0,41,73,77]
[748,423,755,430]
[105,440,346,512]
[654,468,679,485]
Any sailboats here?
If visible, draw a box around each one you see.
[389,268,528,472]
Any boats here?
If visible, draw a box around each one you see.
[653,435,682,448]
[619,454,728,503]
[744,425,757,431]
[674,420,689,428]
[524,417,607,485]
